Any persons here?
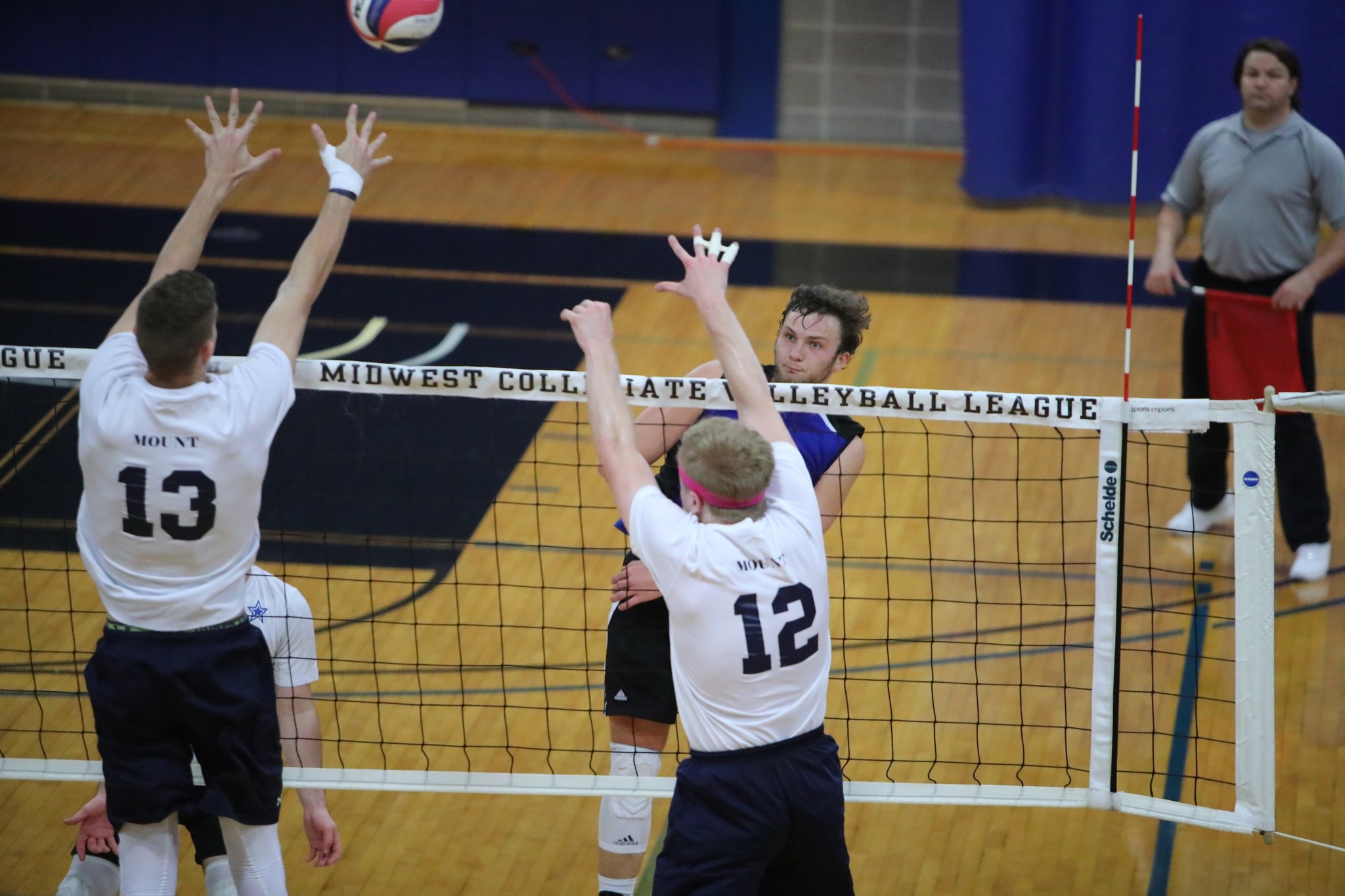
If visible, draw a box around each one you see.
[72,87,395,896]
[595,283,870,895]
[559,226,859,896]
[1145,39,1344,583]
[53,564,343,895]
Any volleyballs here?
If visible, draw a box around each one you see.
[347,0,445,55]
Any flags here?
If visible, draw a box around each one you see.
[1201,288,1305,399]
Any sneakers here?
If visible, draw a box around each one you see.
[1165,490,1234,537]
[1286,541,1332,581]
